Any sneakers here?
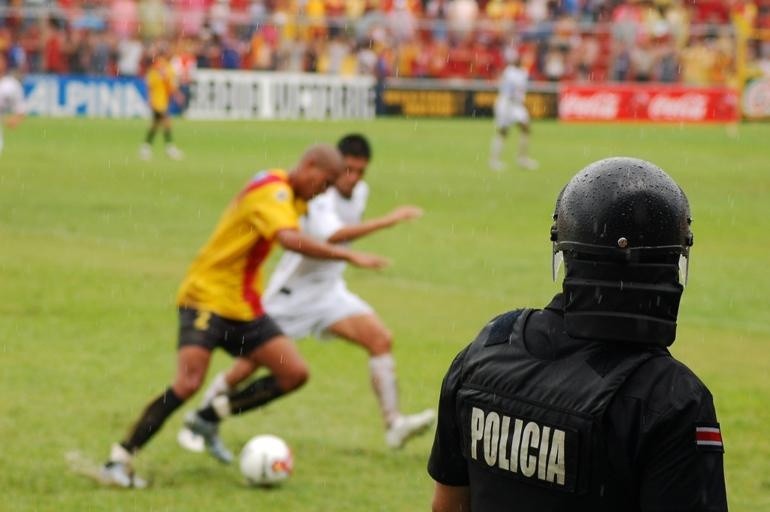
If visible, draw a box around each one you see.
[103,459,148,490]
[384,409,435,450]
[176,411,236,467]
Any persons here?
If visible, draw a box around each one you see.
[176,131,442,468]
[137,39,184,160]
[0,50,28,160]
[78,141,396,493]
[1,1,770,88]
[488,44,543,175]
[426,150,735,512]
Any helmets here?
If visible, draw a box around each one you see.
[553,154,692,283]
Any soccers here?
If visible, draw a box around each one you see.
[237,433,294,486]
